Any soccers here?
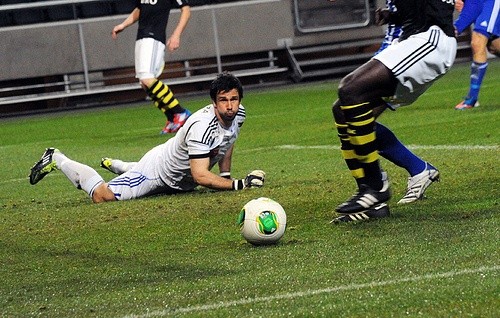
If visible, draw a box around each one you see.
[238,196,287,246]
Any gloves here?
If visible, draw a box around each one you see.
[220,172,231,179]
[232,174,265,190]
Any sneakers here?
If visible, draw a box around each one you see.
[455,99,480,110]
[331,203,391,224]
[100,157,116,174]
[398,162,439,203]
[159,109,191,134]
[30,147,60,185]
[336,179,391,214]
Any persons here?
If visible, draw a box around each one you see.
[112,0,192,134]
[28,73,266,203]
[331,0,464,224]
[452,0,500,110]
